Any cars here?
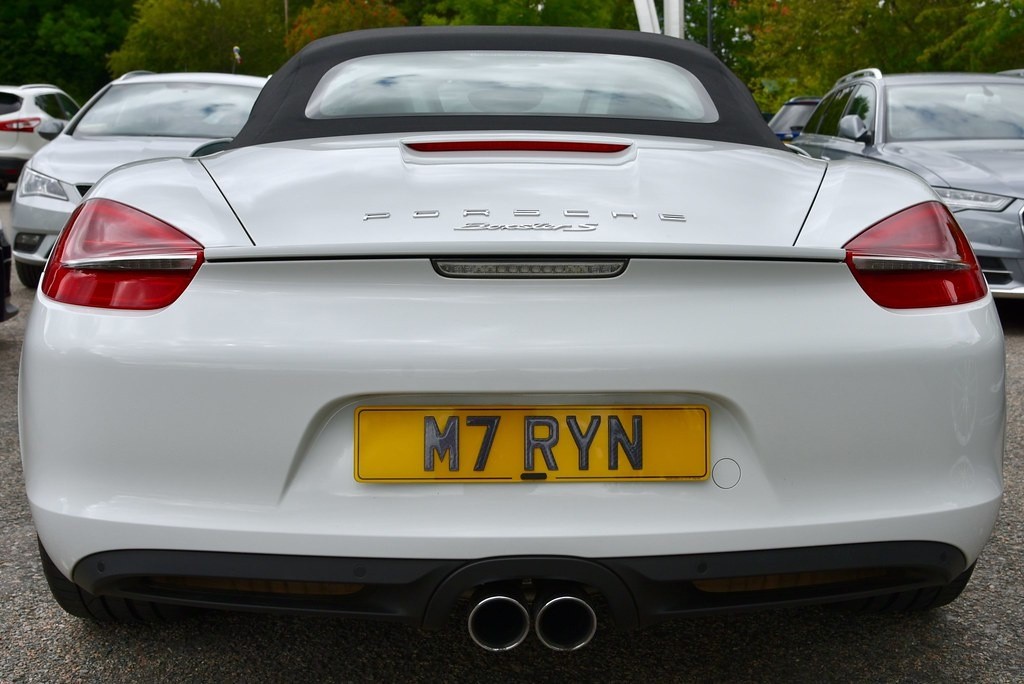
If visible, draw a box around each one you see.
[766,93,823,146]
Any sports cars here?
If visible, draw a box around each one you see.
[18,24,1008,652]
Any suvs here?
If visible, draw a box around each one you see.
[7,67,271,290]
[0,83,80,204]
[786,66,1023,315]
